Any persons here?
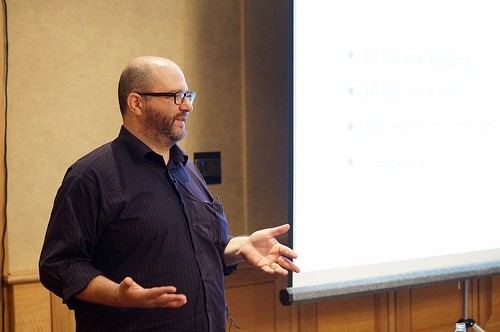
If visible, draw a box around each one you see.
[38,55,299,332]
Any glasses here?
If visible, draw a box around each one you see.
[138,91,196,105]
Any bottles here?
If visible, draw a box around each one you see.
[455,323,467,332]
